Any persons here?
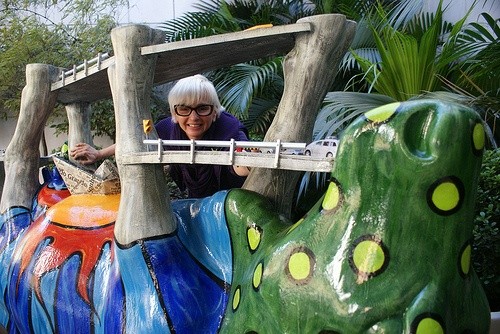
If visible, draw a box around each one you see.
[70,74,258,198]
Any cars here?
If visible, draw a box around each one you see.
[259,148,275,154]
[280,149,304,156]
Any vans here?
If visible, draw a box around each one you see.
[305,139,340,158]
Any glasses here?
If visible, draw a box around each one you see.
[173,104,214,117]
